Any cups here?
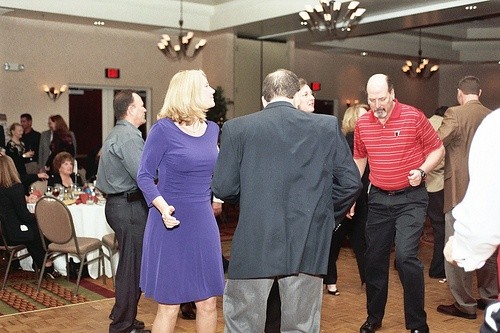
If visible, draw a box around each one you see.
[52,187,60,197]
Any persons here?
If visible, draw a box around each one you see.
[432,75,499,320]
[442,107,500,333]
[420,105,453,280]
[209,66,367,333]
[344,74,447,333]
[0,155,62,282]
[135,68,226,333]
[0,112,87,203]
[96,88,159,333]
[323,102,369,297]
[259,77,318,333]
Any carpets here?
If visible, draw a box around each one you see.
[0,262,145,319]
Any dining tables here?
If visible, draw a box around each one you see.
[16,190,119,279]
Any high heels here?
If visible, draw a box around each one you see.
[326,284,339,296]
[32,261,41,273]
[42,263,62,281]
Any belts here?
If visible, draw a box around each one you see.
[370,183,425,195]
[108,192,124,196]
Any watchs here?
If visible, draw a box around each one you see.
[417,168,427,181]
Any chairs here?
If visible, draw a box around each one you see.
[35,196,107,294]
[97,230,119,290]
[0,229,40,290]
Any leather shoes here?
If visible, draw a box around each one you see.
[108,314,151,333]
[360,322,382,333]
[476,299,487,309]
[437,305,477,319]
[411,324,428,333]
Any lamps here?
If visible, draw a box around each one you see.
[402,27,440,80]
[299,0,366,34]
[42,84,67,102]
[157,1,207,62]
